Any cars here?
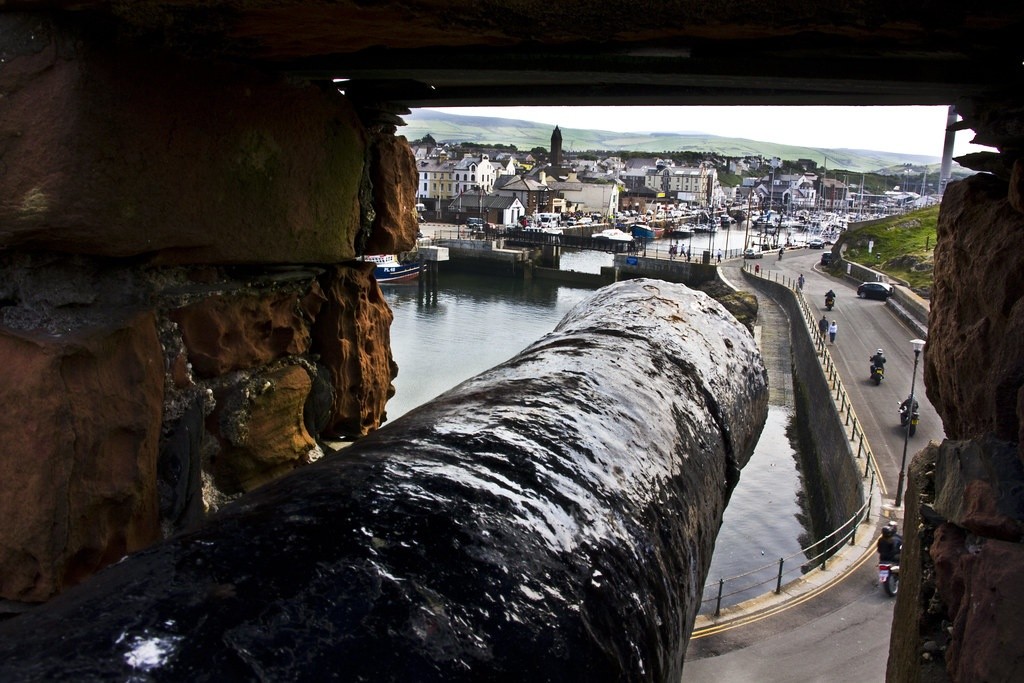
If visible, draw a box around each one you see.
[857,282,894,300]
[567,206,706,224]
[744,247,764,259]
[821,252,832,266]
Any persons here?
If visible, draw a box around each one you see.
[755,262,760,277]
[869,349,886,380]
[828,320,837,346]
[899,393,919,426]
[778,247,784,261]
[818,314,829,343]
[669,243,722,264]
[798,274,805,291]
[877,521,902,565]
[824,289,836,306]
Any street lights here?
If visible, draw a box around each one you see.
[896,337,926,505]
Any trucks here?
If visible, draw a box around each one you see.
[540,213,562,228]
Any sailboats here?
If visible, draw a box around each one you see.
[708,164,937,248]
[682,175,717,233]
[592,158,634,247]
[631,172,665,240]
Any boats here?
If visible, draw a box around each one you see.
[672,227,695,238]
[361,255,427,283]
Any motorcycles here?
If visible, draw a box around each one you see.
[897,401,919,437]
[825,292,834,310]
[870,356,884,385]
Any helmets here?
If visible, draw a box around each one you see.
[888,521,899,532]
[881,525,891,536]
[908,394,915,399]
[877,349,883,353]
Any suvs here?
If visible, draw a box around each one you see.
[466,218,488,228]
[809,238,825,249]
